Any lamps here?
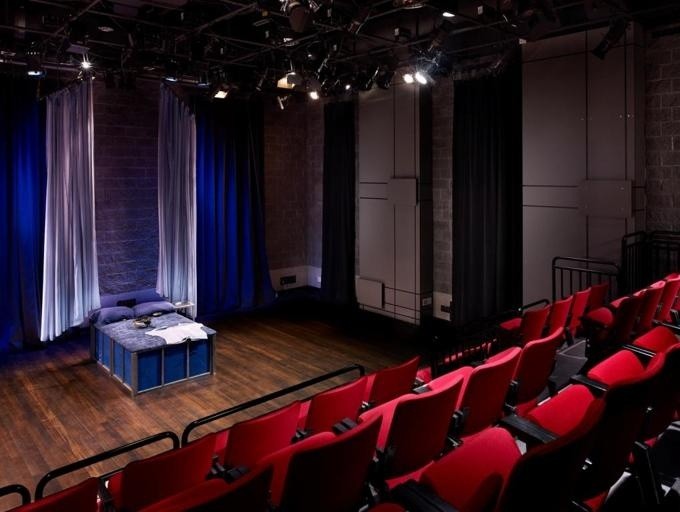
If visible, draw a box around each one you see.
[207,59,232,101]
[21,33,48,79]
[253,1,530,112]
